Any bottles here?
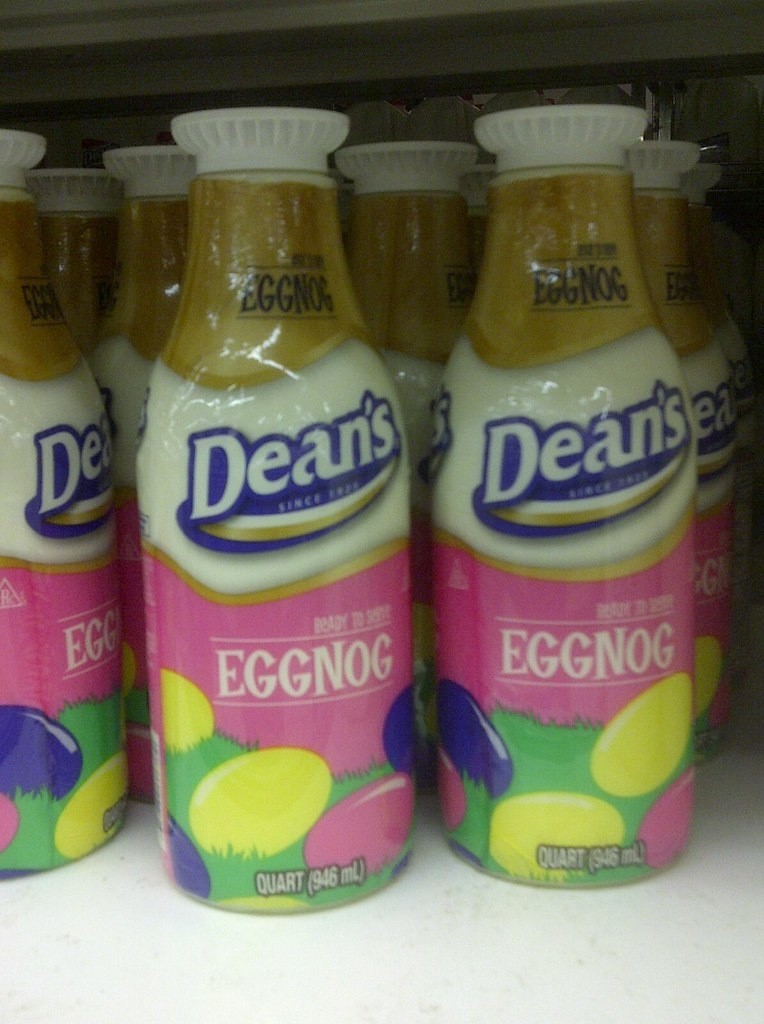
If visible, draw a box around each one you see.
[0,100,762,918]
